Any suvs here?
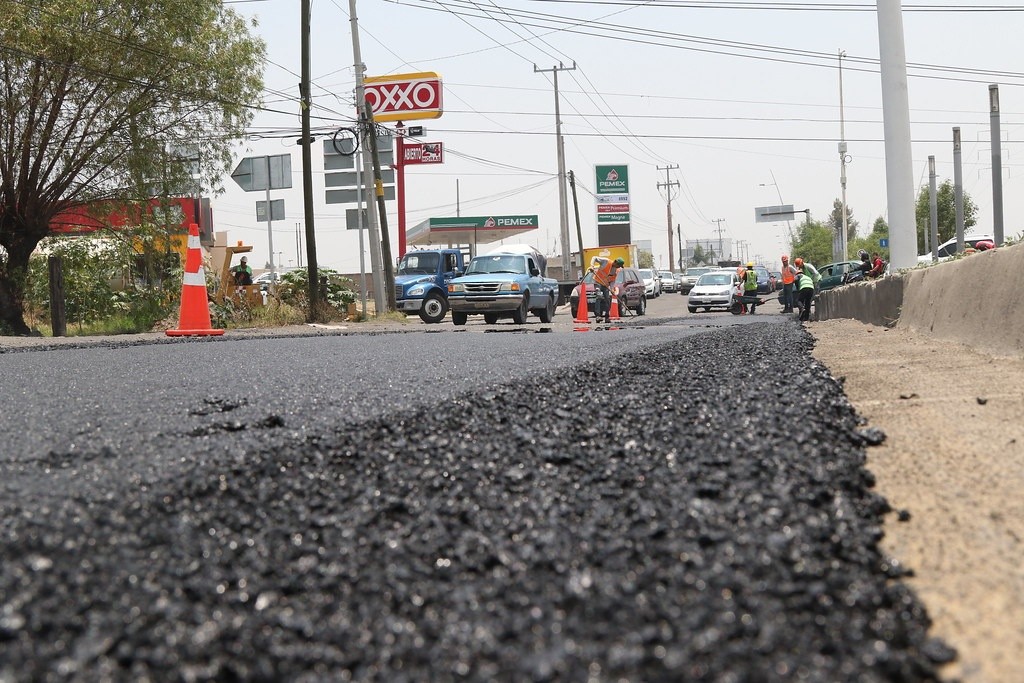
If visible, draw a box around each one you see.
[917,234,994,264]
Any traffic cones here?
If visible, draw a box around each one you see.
[573,282,591,323]
[166,223,225,336]
[609,294,620,320]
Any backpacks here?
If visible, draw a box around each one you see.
[881,261,887,274]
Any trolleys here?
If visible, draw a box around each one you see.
[731,293,778,315]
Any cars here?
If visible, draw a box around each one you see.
[657,271,678,293]
[674,266,721,296]
[687,271,745,313]
[252,271,279,290]
[720,266,783,294]
[638,269,661,299]
[778,260,864,305]
[570,267,646,318]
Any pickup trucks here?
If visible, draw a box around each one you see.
[448,253,559,326]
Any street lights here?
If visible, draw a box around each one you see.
[952,126,965,254]
[988,85,1004,247]
[928,155,938,262]
[760,184,793,235]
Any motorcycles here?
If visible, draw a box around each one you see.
[836,265,887,287]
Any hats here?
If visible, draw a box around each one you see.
[616,257,625,268]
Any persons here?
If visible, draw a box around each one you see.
[589,255,624,323]
[870,252,882,277]
[795,257,822,294]
[780,255,797,313]
[795,270,814,321]
[738,262,758,315]
[848,252,872,281]
[228,256,252,286]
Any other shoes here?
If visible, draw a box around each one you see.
[750,311,754,315]
[803,315,809,320]
[605,319,611,323]
[780,310,788,313]
[596,317,603,323]
[788,309,793,313]
[799,309,807,320]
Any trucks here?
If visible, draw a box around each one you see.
[384,248,466,323]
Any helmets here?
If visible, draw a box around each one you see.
[782,255,788,261]
[794,258,803,267]
[795,270,802,274]
[746,262,754,267]
[861,253,869,262]
[240,256,248,263]
